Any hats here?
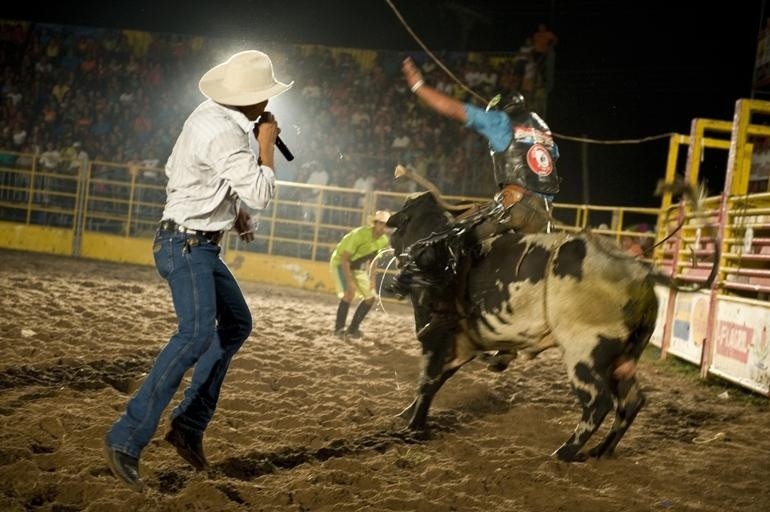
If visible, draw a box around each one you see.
[368,210,392,233]
[199,49,294,106]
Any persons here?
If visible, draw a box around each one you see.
[622,230,643,259]
[231,30,553,257]
[104,49,295,486]
[1,29,218,234]
[329,211,390,336]
[403,57,561,343]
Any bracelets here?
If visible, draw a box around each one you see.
[410,80,424,93]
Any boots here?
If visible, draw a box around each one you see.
[417,301,454,341]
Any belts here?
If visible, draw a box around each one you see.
[158,220,226,243]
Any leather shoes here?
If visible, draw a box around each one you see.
[104,445,139,486]
[166,432,208,471]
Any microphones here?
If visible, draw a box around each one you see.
[258,117,295,161]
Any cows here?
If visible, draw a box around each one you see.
[385,178,721,464]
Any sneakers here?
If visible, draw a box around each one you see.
[488,351,515,371]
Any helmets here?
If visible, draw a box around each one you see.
[485,92,526,116]
